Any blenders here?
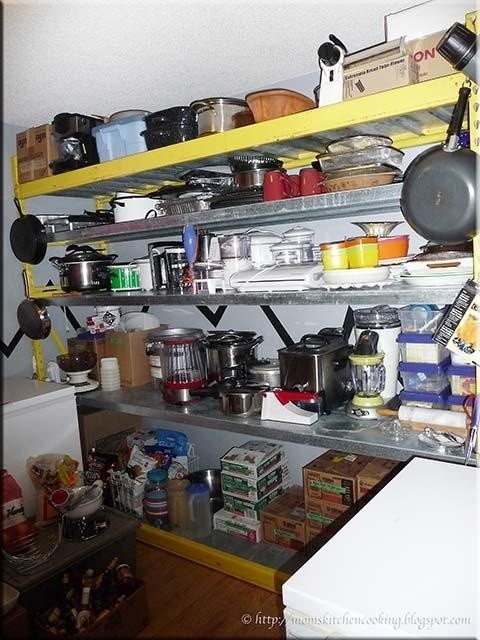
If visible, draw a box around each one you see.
[160,335,205,406]
[342,351,387,420]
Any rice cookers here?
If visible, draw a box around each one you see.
[245,227,281,266]
[105,260,137,292]
[130,239,183,292]
[109,194,165,222]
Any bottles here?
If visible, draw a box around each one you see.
[143,467,169,525]
[74,587,93,636]
[40,588,76,629]
[95,555,119,592]
[436,21,477,82]
[59,572,72,587]
[77,568,97,611]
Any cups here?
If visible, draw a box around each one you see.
[299,166,324,196]
[286,173,300,198]
[259,167,294,203]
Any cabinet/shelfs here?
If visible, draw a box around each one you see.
[10,72,476,593]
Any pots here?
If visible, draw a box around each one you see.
[249,357,280,386]
[120,313,161,333]
[189,378,269,418]
[396,82,479,246]
[187,95,248,134]
[202,329,263,381]
[48,244,119,294]
[5,298,51,357]
[7,197,47,268]
[146,327,207,384]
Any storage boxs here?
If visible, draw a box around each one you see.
[449,365,476,395]
[212,439,406,558]
[90,111,148,163]
[341,28,459,101]
[448,395,475,413]
[66,337,103,381]
[0,608,28,639]
[15,124,55,184]
[32,579,150,640]
[103,324,170,386]
[398,334,450,366]
[400,364,450,394]
[400,391,448,406]
[396,304,448,338]
[112,196,169,222]
[2,505,141,602]
[77,405,144,464]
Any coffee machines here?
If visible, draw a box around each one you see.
[49,112,104,174]
[191,230,251,291]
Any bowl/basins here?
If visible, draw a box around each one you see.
[100,357,122,391]
[321,240,348,271]
[179,467,222,511]
[347,238,378,268]
[225,165,273,191]
[63,482,105,519]
[246,89,317,123]
